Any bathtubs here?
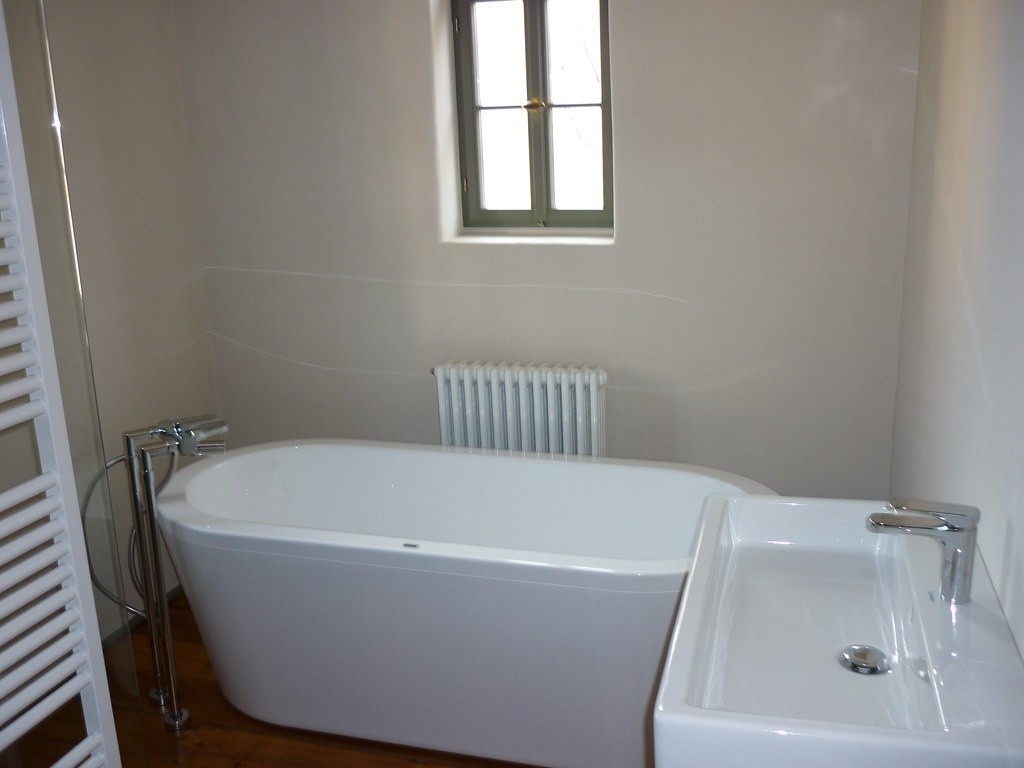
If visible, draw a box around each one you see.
[151,433,781,768]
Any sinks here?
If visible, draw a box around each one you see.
[651,490,1024,768]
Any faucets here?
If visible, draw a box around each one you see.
[863,496,983,606]
[122,412,230,712]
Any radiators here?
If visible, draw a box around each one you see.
[431,356,609,456]
